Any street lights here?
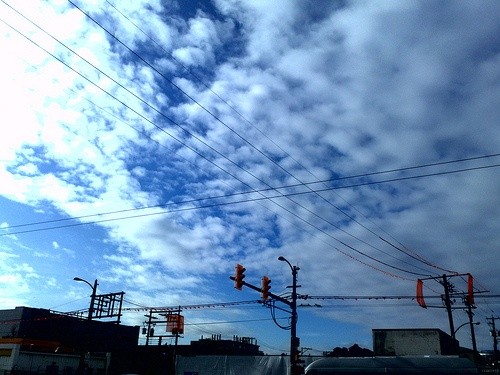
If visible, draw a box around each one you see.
[73,275,98,375]
[277,256,299,373]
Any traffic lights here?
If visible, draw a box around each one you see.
[235,263,246,290]
[263,277,272,301]
